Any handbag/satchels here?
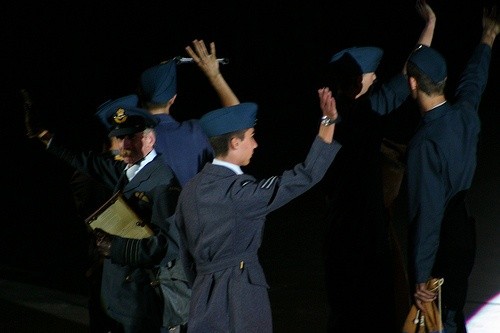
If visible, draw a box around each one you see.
[401,277,444,333]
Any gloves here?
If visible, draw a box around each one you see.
[19,89,48,137]
[93,227,117,259]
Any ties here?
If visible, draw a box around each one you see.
[113,168,129,196]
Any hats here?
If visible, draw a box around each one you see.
[105,105,160,136]
[95,94,138,129]
[406,43,447,83]
[136,59,177,104]
[332,46,385,76]
[199,102,258,137]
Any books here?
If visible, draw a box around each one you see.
[85,190,154,240]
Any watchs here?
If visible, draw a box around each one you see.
[321,116,334,127]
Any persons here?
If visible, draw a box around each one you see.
[311,0,436,333]
[405,3,500,333]
[134,39,242,188]
[25,95,182,333]
[158,86,343,333]
